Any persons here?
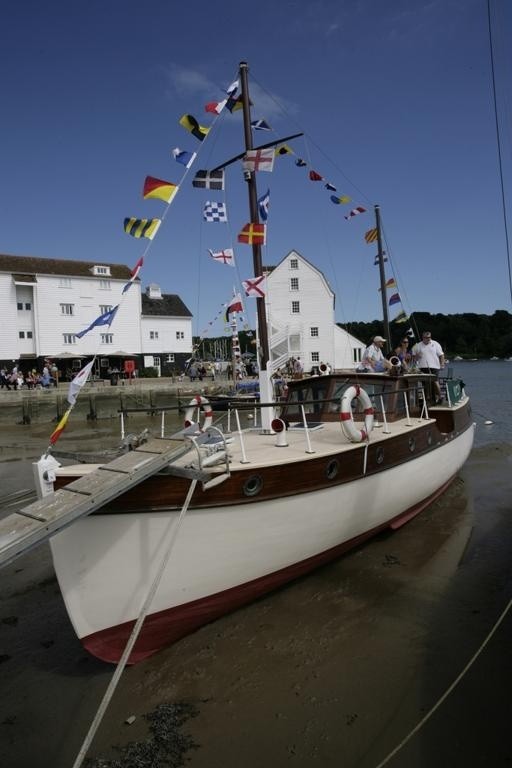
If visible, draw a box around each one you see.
[1,366,54,391]
[412,331,444,403]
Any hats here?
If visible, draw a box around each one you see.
[373,335,387,342]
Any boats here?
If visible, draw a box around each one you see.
[35,54,478,667]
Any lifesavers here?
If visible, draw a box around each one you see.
[340,386,374,443]
[185,395,213,435]
